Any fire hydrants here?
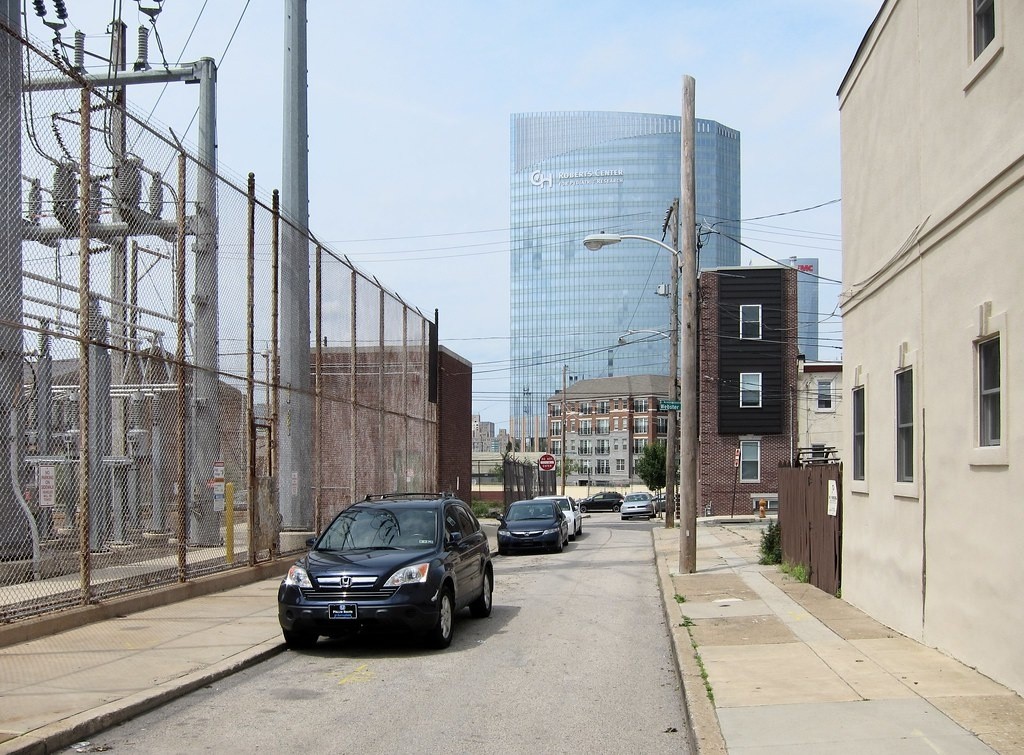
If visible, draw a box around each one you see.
[759,496,767,518]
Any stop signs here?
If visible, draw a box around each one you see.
[539,453,555,470]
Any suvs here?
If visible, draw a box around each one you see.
[277,491,494,651]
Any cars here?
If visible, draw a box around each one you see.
[495,499,570,554]
[638,491,674,512]
[526,494,583,542]
[619,492,657,520]
[576,490,624,513]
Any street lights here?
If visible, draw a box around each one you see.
[583,231,698,574]
[617,329,678,529]
